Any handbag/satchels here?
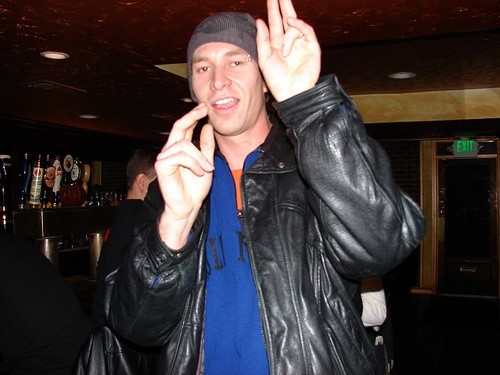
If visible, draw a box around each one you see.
[72,278,153,375]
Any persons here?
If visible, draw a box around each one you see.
[0,229,95,375]
[94,144,162,330]
[104,0,427,375]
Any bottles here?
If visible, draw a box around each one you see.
[28,154,44,209]
[17,153,31,209]
[51,156,63,208]
[88,191,127,206]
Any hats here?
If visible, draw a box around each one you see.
[187,12,271,103]
